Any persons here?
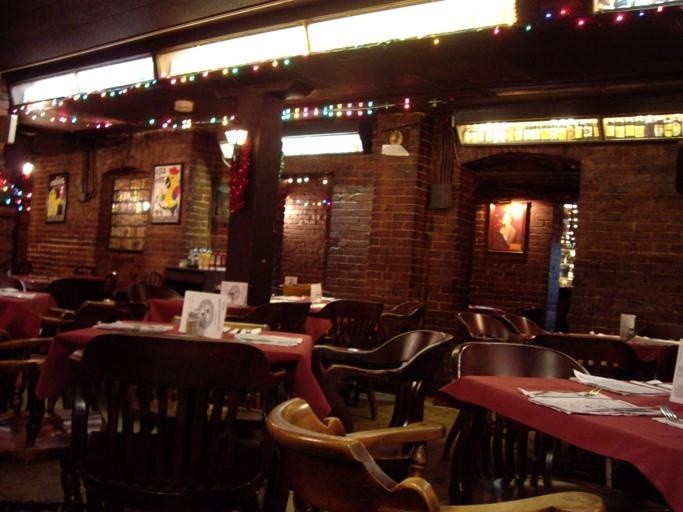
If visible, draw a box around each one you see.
[497,212,516,250]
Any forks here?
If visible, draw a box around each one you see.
[659,406,683,425]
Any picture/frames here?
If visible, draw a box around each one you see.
[485,200,530,257]
[152,162,183,224]
[45,172,69,224]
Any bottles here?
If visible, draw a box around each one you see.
[193,247,227,268]
[186,311,199,336]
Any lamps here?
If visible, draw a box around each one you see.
[219,125,249,169]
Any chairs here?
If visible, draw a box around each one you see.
[0,263,683,512]
